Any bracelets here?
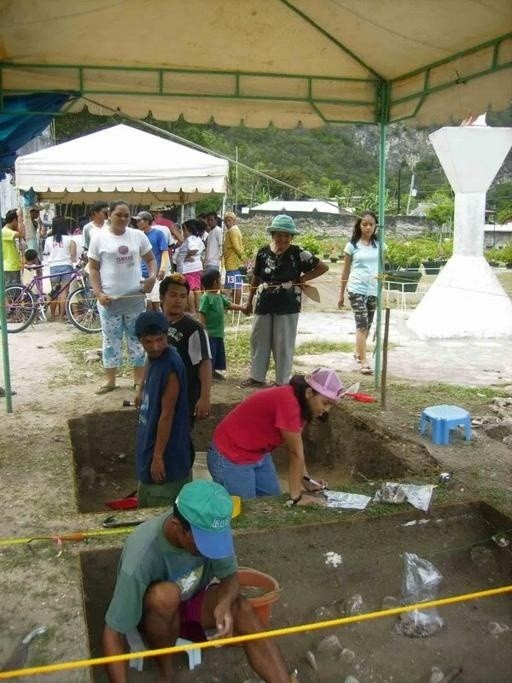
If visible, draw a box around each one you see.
[283,495,303,508]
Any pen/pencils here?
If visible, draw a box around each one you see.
[304,476,330,491]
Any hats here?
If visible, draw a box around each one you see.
[304,367,346,402]
[175,480,235,560]
[130,211,154,221]
[266,214,301,235]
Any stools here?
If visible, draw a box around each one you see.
[417,403,473,448]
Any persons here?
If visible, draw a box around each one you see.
[132,310,194,511]
[99,479,292,683]
[0,196,253,396]
[239,211,331,387]
[204,367,346,509]
[336,210,390,376]
[158,269,214,471]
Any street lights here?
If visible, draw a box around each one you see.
[394,160,407,215]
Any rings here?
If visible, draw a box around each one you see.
[205,411,209,416]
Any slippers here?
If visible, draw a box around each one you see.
[93,384,120,394]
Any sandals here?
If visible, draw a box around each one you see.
[353,351,375,375]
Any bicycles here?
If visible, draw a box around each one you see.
[4,245,104,333]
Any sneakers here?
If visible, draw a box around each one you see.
[212,370,226,383]
[239,376,267,387]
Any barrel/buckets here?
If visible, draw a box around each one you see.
[235,566,283,629]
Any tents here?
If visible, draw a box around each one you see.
[13,123,229,272]
